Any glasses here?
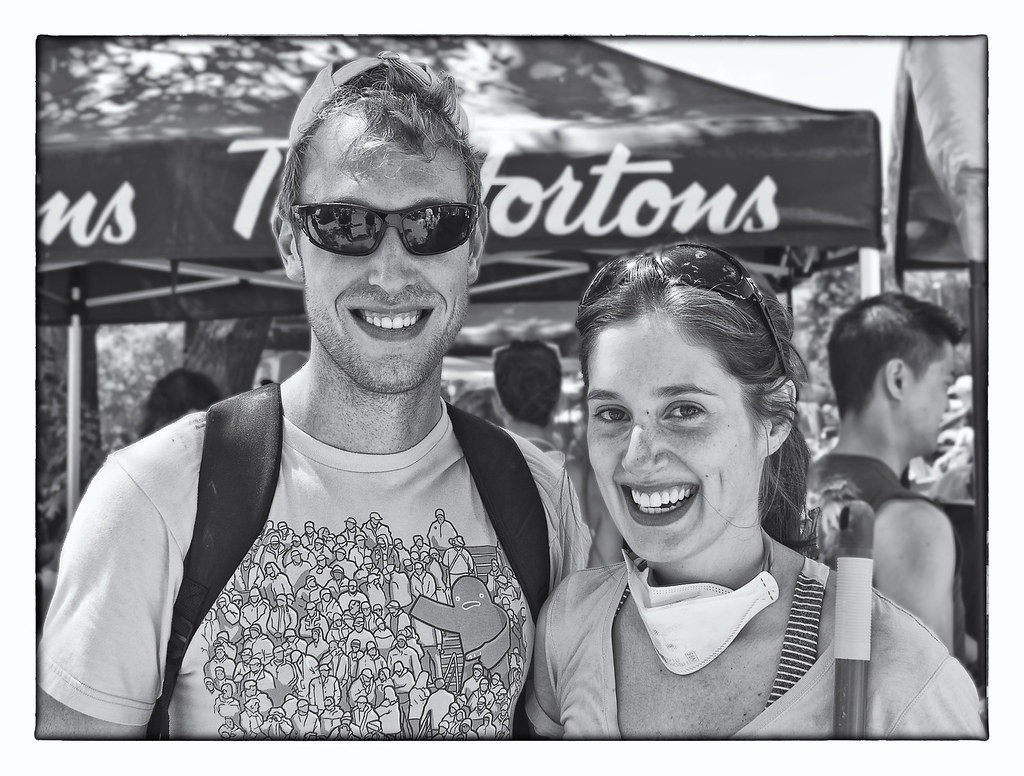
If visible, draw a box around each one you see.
[578,244,791,375]
[287,200,482,256]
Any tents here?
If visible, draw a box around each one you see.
[34,35,877,539]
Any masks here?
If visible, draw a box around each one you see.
[622,548,779,676]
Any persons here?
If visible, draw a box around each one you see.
[486,339,627,570]
[132,365,217,441]
[790,292,974,677]
[521,243,986,738]
[36,52,600,736]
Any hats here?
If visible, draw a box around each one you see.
[271,50,470,157]
